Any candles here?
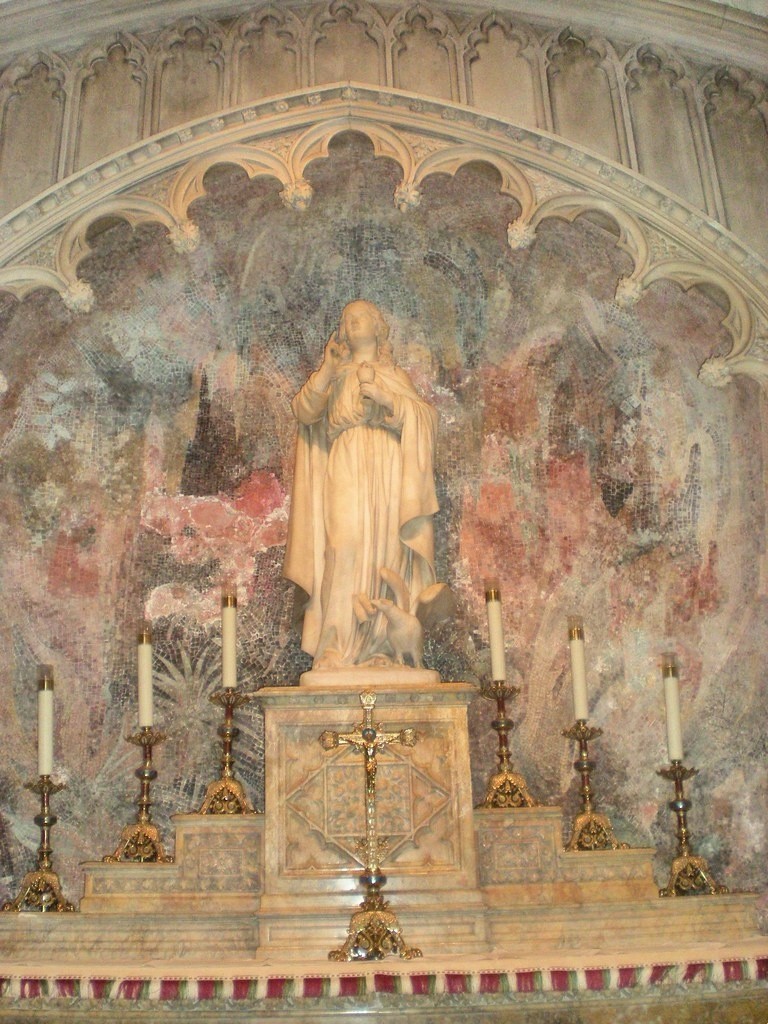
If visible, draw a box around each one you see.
[136,618,155,728]
[661,651,684,762]
[483,577,507,680]
[220,581,239,688]
[566,615,590,720]
[36,663,54,776]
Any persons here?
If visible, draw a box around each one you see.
[284,300,441,664]
